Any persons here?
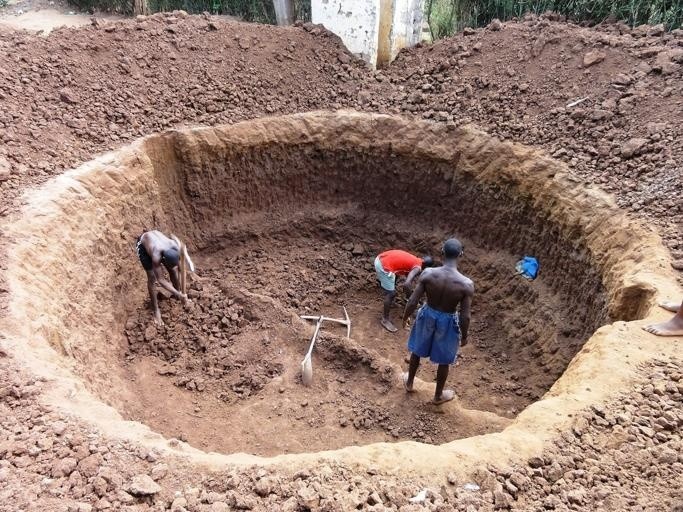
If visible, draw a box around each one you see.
[402,238,476,406]
[374,250,433,332]
[641,296,683,336]
[137,230,188,326]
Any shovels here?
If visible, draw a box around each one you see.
[301,315,324,384]
